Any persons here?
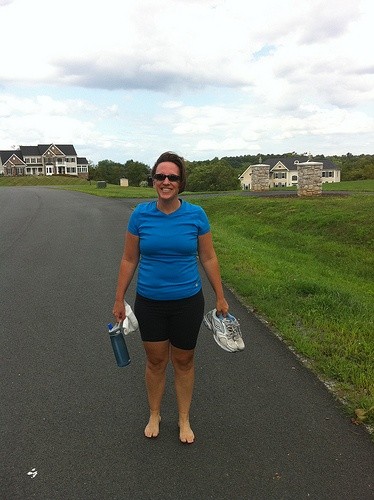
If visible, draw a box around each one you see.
[112,151,230,444]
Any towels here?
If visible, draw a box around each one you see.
[122,300,138,336]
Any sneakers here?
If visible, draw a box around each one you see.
[204,309,245,354]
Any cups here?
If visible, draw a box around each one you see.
[108,327,131,367]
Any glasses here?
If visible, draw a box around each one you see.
[154,173,179,183]
